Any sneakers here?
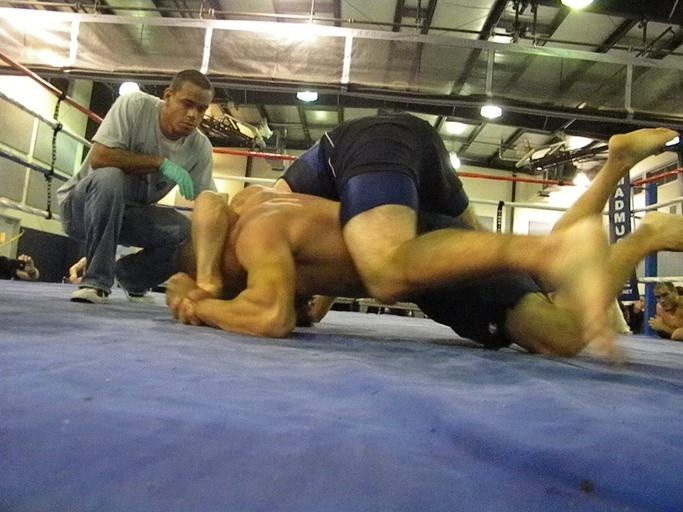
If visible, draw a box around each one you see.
[71,287,111,304]
[115,271,155,304]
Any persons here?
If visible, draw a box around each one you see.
[170,113,682,358]
[68,255,88,283]
[648,282,683,341]
[14,253,40,280]
[619,295,645,335]
[58,69,220,303]
[165,184,682,358]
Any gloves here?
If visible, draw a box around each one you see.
[159,157,194,201]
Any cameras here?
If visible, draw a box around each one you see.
[0,256,26,280]
